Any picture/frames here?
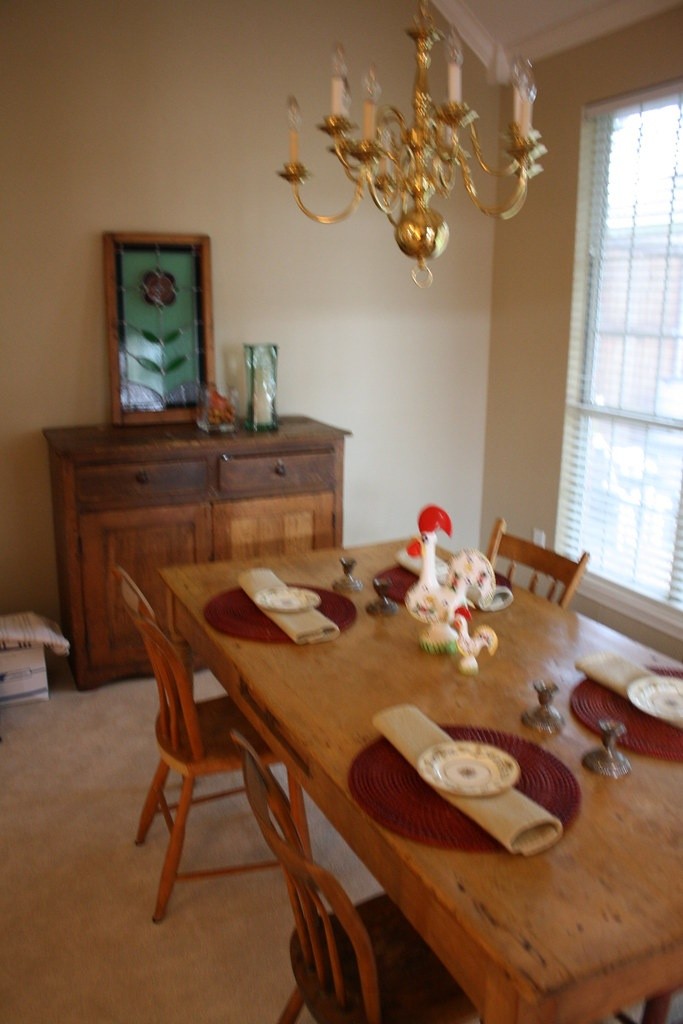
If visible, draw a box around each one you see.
[104,225,212,425]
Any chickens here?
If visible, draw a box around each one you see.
[451,605,499,661]
[406,505,496,633]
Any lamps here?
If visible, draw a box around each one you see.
[283,0,551,292]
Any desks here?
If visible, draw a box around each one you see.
[153,538,683,1024]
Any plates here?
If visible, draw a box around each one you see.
[254,587,320,611]
[627,676,683,729]
[418,741,521,796]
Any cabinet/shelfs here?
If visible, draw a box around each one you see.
[44,415,353,695]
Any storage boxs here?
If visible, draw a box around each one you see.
[0,618,50,706]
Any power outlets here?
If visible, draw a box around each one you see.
[531,528,546,546]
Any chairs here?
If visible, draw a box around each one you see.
[223,728,483,1024]
[111,563,313,924]
[485,519,592,606]
